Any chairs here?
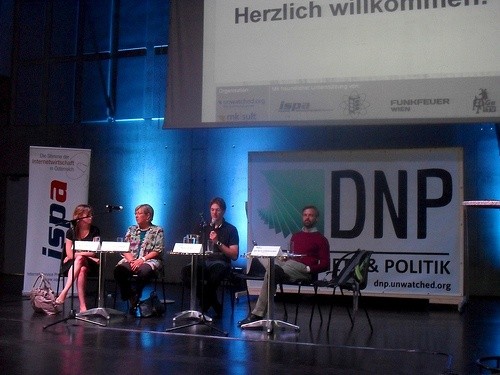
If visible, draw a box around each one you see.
[56,243,75,297]
[277,273,323,327]
[151,269,167,312]
[309,250,373,333]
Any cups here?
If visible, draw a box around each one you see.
[93,237,101,242]
[117,237,123,242]
[286,242,294,255]
[206,240,214,252]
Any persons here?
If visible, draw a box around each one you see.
[237,206,330,326]
[181,197,239,318]
[52,204,103,313]
[113,204,165,317]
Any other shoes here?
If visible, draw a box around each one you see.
[126,306,138,318]
[133,294,140,307]
[55,296,67,304]
[194,306,223,322]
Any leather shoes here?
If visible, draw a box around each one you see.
[238,313,263,325]
[274,265,290,283]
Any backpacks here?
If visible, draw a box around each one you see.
[333,250,370,290]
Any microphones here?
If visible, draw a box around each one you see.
[105,204,124,210]
[212,217,216,232]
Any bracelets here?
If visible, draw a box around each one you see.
[140,257,146,262]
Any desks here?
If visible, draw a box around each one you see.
[74,250,130,320]
[166,250,216,322]
[241,252,306,333]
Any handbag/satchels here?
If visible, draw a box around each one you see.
[30,271,58,316]
[134,291,164,320]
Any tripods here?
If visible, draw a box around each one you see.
[41,210,107,329]
[165,225,228,335]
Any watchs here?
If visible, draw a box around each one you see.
[216,241,222,247]
